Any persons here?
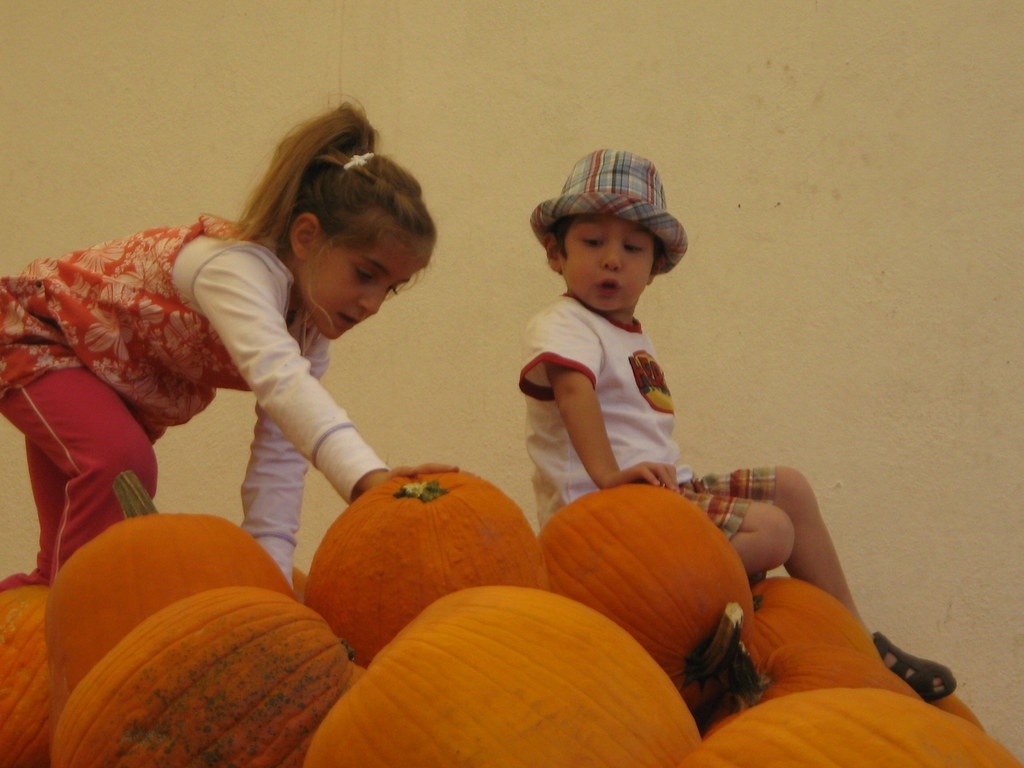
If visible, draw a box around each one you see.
[0,92,461,594]
[516,148,959,703]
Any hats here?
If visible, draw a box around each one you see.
[531,150,687,275]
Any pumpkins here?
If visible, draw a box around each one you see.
[0,471,1024,768]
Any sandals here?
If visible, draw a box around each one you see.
[874,628,956,702]
